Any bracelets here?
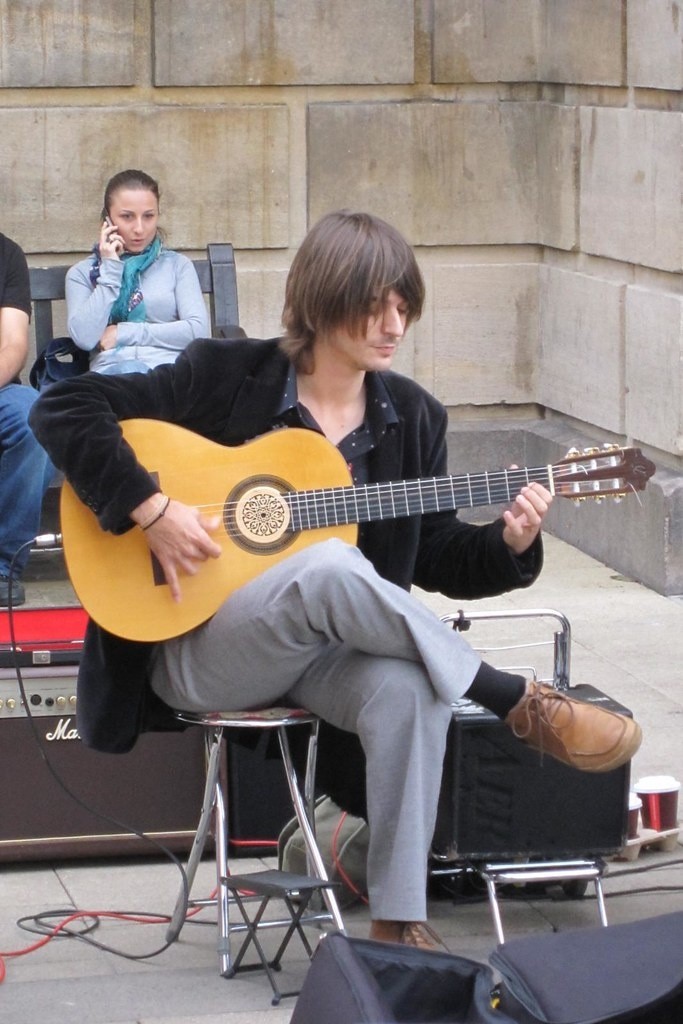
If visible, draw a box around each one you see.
[140,495,171,531]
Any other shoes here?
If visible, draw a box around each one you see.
[0,574,25,606]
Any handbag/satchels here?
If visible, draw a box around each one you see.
[29,336,90,390]
[288,911,683,1024]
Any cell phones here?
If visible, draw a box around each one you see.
[100,207,117,236]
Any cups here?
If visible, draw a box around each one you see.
[629,793,643,839]
[634,776,680,832]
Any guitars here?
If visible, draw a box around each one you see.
[59,416,658,644]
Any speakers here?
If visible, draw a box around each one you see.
[429,684,633,864]
[225,724,326,857]
[0,666,217,865]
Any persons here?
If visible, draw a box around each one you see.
[0,233,55,606]
[62,170,214,375]
[25,209,644,955]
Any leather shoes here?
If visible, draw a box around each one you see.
[371,919,451,953]
[506,682,643,776]
